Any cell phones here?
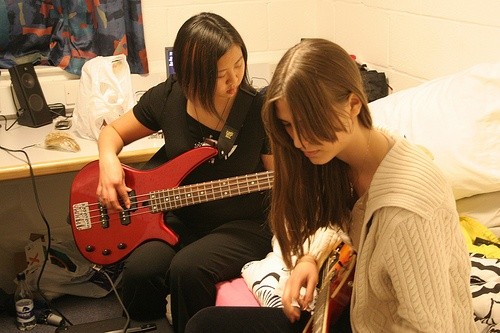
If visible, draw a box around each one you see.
[55,120,72,130]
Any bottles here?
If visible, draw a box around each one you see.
[8,293,64,327]
[14,272,36,332]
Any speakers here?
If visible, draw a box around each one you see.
[8,63,53,129]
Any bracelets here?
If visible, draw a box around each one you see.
[295,259,317,266]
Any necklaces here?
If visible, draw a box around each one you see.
[194,98,230,142]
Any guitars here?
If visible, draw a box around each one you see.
[303,240,356,333]
[70,147,275,266]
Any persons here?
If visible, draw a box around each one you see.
[95,13,277,333]
[185,37,480,333]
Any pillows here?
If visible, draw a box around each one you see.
[366,58,500,201]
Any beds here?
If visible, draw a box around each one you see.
[215,190,499,333]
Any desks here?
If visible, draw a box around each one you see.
[0,112,159,178]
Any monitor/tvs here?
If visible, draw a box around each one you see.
[165,47,176,78]
